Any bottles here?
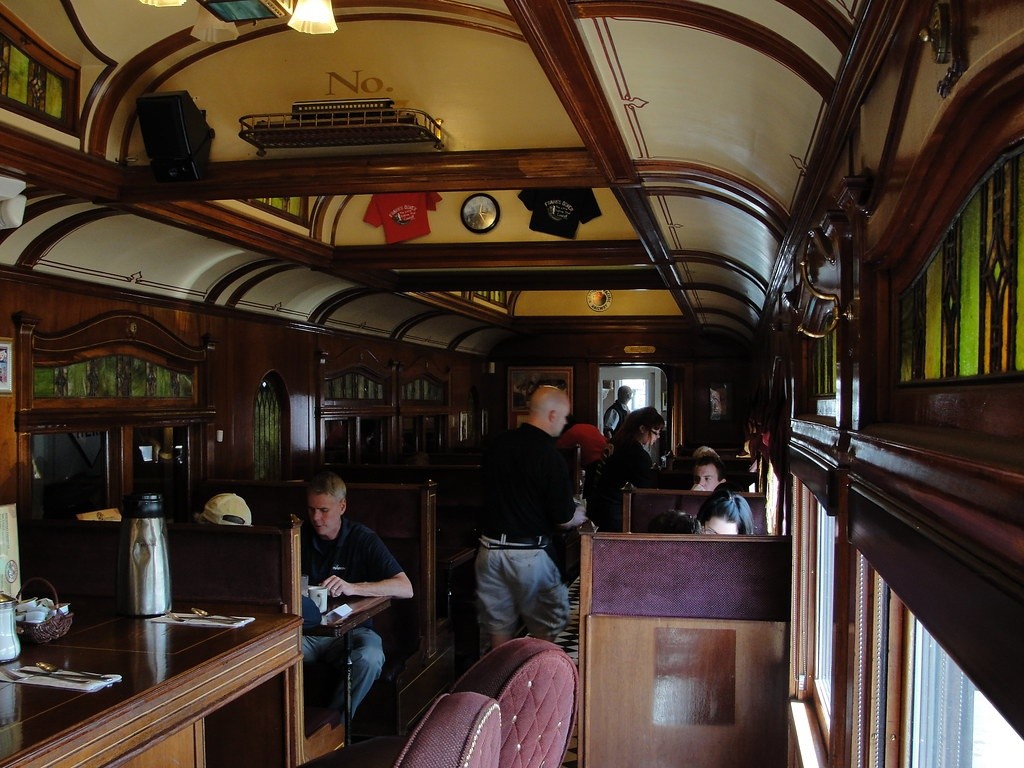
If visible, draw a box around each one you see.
[0,591,21,663]
[576,470,586,501]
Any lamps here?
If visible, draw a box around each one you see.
[139,0,339,43]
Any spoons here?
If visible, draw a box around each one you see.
[191,608,248,620]
[36,662,105,677]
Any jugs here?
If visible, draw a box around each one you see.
[128,492,173,616]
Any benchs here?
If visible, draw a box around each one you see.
[28,460,483,759]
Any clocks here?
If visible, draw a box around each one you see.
[461,193,501,234]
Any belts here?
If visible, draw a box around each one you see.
[481,530,548,545]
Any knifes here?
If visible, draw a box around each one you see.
[17,670,112,681]
[165,617,236,621]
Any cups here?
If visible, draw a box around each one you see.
[691,483,705,490]
[309,586,328,613]
[300,574,308,598]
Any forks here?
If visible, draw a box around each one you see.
[0,667,88,683]
[165,610,236,624]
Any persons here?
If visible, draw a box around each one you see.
[696,491,754,535]
[583,408,665,531]
[301,473,413,749]
[689,447,743,492]
[647,510,701,534]
[603,387,631,441]
[198,496,321,630]
[556,415,606,474]
[466,386,587,649]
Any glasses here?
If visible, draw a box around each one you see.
[703,528,717,535]
[646,428,661,436]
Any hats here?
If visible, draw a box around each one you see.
[693,446,720,460]
[201,493,253,527]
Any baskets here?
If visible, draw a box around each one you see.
[15,577,73,645]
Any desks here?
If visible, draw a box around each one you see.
[302,595,393,747]
[0,596,305,768]
[436,544,478,625]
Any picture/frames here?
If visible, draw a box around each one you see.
[0,336,16,398]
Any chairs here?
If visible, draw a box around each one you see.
[394,693,502,767]
[451,638,581,767]
[577,445,789,619]
